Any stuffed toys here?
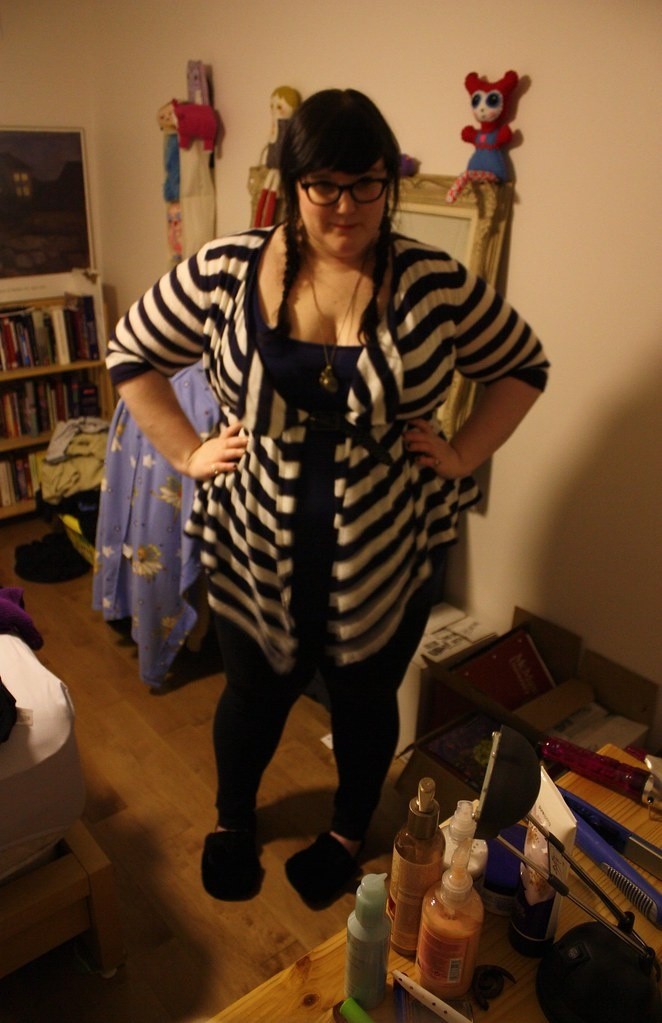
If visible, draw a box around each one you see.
[445,70,519,203]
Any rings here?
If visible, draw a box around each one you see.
[432,459,440,468]
[210,461,217,475]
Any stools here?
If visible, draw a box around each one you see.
[0,813,129,987]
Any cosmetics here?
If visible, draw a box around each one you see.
[346,765,578,1008]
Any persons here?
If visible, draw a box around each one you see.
[253,86,301,228]
[105,89,552,906]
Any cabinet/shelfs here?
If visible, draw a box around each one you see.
[0,269,117,519]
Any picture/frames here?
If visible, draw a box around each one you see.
[277,174,512,441]
[0,126,97,278]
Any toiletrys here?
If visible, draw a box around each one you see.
[386,777,446,958]
[441,800,490,880]
[345,872,392,1008]
[413,837,485,999]
[507,765,578,959]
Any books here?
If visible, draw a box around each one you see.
[422,625,557,792]
[0,296,100,507]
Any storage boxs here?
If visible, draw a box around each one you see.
[390,603,656,819]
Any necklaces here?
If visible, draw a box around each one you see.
[306,256,365,392]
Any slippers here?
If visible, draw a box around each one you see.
[202,824,258,902]
[285,830,363,891]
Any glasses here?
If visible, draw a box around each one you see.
[296,170,392,205]
